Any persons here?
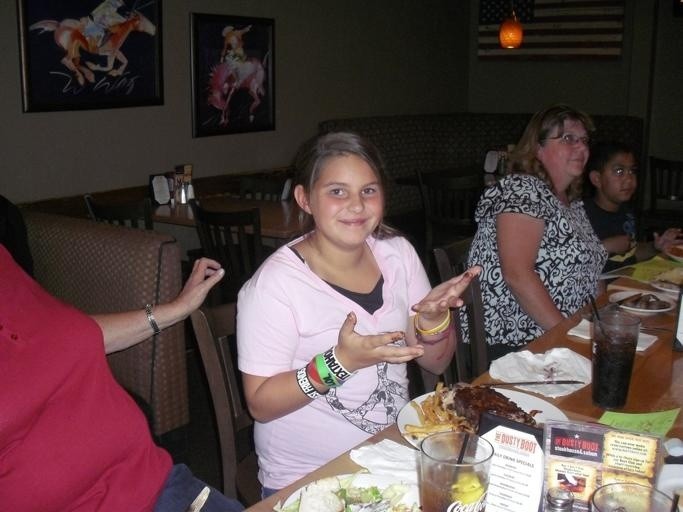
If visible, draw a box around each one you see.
[577,136,683,285]
[233,133,486,502]
[1,245,245,512]
[219,23,253,62]
[467,103,610,371]
[91,1,124,47]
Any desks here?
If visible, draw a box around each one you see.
[245,265,683,512]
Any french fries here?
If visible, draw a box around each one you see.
[404,381,474,439]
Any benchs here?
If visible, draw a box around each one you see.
[314,108,642,238]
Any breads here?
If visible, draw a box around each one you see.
[298,475,344,512]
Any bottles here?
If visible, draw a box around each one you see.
[544,488,575,512]
[498,152,514,174]
[175,180,197,205]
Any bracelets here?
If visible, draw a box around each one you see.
[297,347,353,399]
[144,302,160,336]
[413,308,456,344]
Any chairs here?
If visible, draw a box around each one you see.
[18,171,313,504]
[415,170,485,391]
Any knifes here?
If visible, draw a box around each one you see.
[484,380,585,387]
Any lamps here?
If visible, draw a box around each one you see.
[496,1,523,51]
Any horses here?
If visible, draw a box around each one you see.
[28,4,156,86]
[207,30,268,124]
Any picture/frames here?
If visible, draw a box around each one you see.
[15,1,166,114]
[186,10,277,140]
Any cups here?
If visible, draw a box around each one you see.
[417,432,494,512]
[589,482,681,512]
[591,311,641,412]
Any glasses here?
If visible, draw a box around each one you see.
[541,135,589,146]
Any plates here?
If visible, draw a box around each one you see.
[609,290,678,317]
[397,384,571,464]
[278,474,421,512]
